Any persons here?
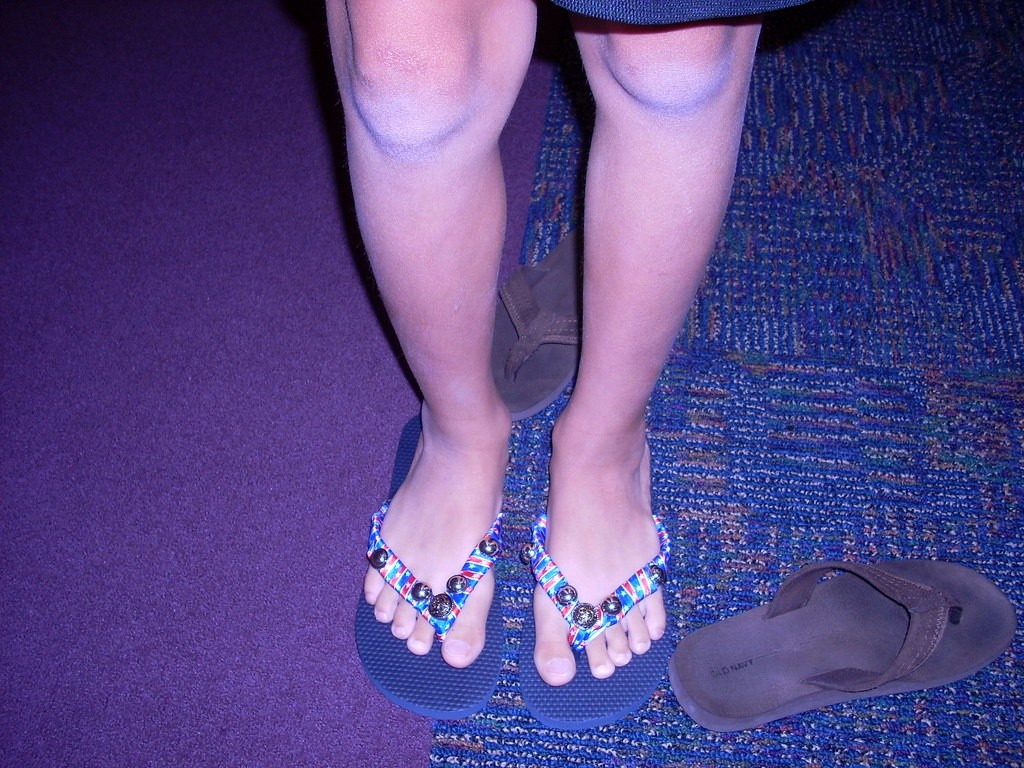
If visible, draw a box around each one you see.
[324,0,806,730]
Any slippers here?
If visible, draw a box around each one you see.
[355,414,504,720]
[491,223,583,422]
[668,560,1017,732]
[518,513,671,730]
